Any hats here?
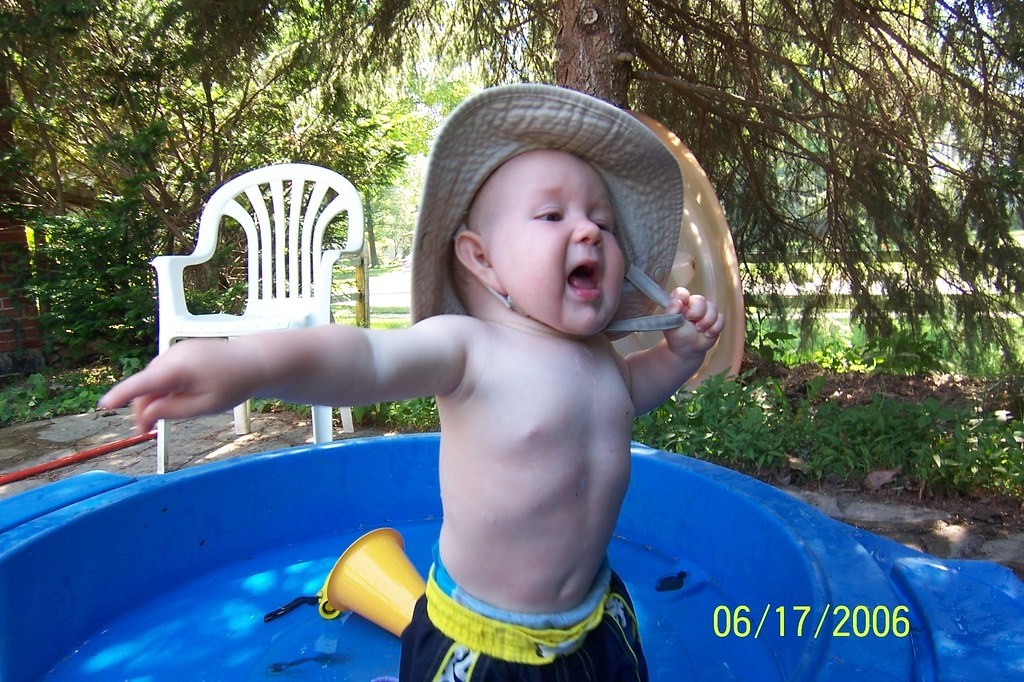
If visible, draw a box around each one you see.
[411,84,686,335]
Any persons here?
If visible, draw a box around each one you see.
[100,84,726,682]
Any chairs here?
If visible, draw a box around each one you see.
[150,163,366,474]
